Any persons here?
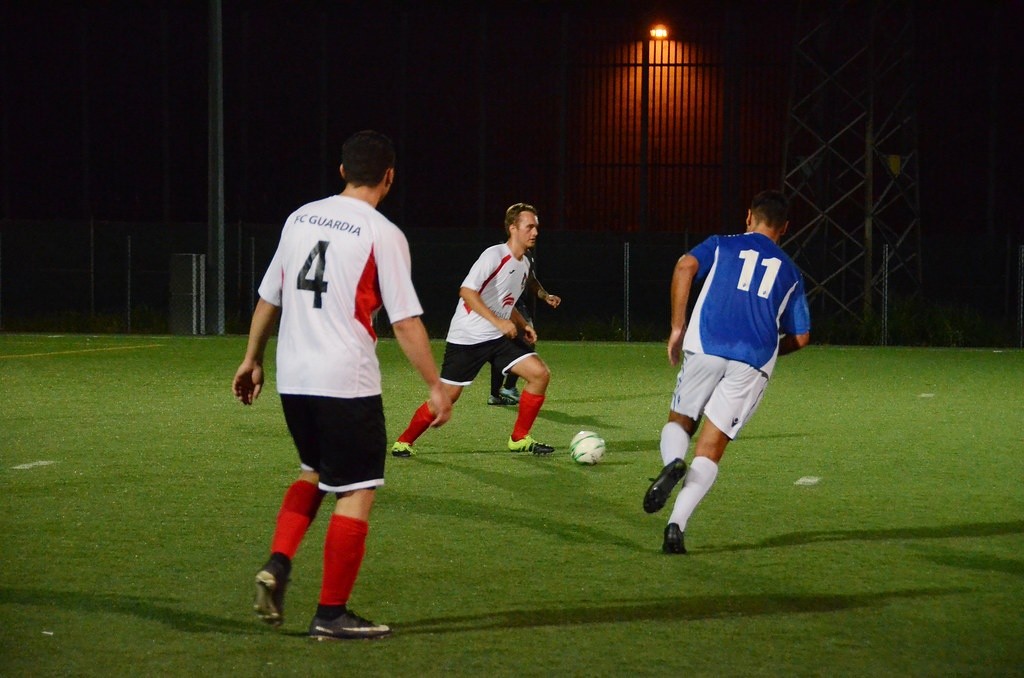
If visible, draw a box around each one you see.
[487,249,562,405]
[232,130,451,642]
[391,203,554,457]
[642,191,812,554]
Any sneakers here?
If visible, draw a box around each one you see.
[254,560,289,624]
[664,523,688,553]
[488,394,505,404]
[391,442,414,457]
[309,610,392,637]
[499,387,520,401]
[642,459,688,514]
[507,434,555,454]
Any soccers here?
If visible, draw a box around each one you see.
[569,430,606,466]
